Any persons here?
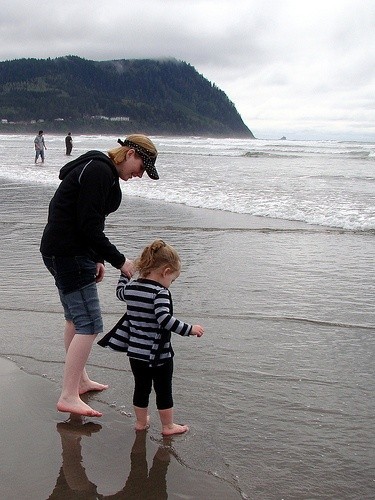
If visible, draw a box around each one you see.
[97,239,205,435]
[40,134,159,416]
[65,132,73,155]
[34,130,47,164]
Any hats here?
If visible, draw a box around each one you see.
[117,137,160,181]
